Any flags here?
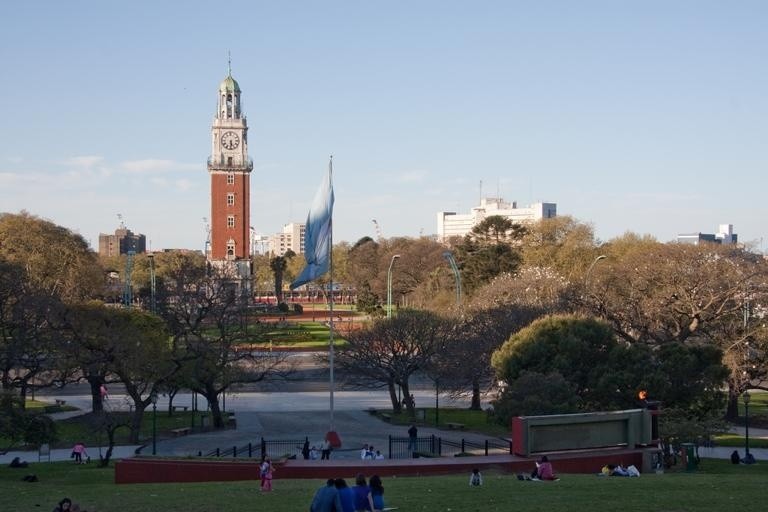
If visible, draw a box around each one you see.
[287,156,336,293]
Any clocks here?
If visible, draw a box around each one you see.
[220,131,239,150]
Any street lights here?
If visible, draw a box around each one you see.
[743,386,750,463]
[386,255,400,318]
[743,296,755,374]
[585,255,608,288]
[147,253,156,321]
[442,252,462,303]
[150,387,158,455]
[124,249,136,307]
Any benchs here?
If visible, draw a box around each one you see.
[54,398,68,405]
[446,422,466,431]
[173,405,189,412]
[171,426,190,437]
[401,401,416,407]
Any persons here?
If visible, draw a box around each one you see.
[595,462,641,477]
[367,474,384,511]
[9,456,25,467]
[350,472,381,512]
[257,455,269,492]
[360,443,369,459]
[333,477,359,512]
[80,455,91,464]
[263,458,276,492]
[468,467,482,487]
[309,478,344,512]
[730,449,740,464]
[373,449,385,460]
[404,422,417,450]
[312,441,322,459]
[366,446,376,460]
[301,440,314,459]
[68,503,81,512]
[320,437,332,460]
[72,442,88,464]
[525,453,542,481]
[52,496,71,512]
[535,455,560,482]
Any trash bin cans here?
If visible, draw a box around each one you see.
[202,415,209,430]
[681,443,694,468]
[229,418,236,430]
